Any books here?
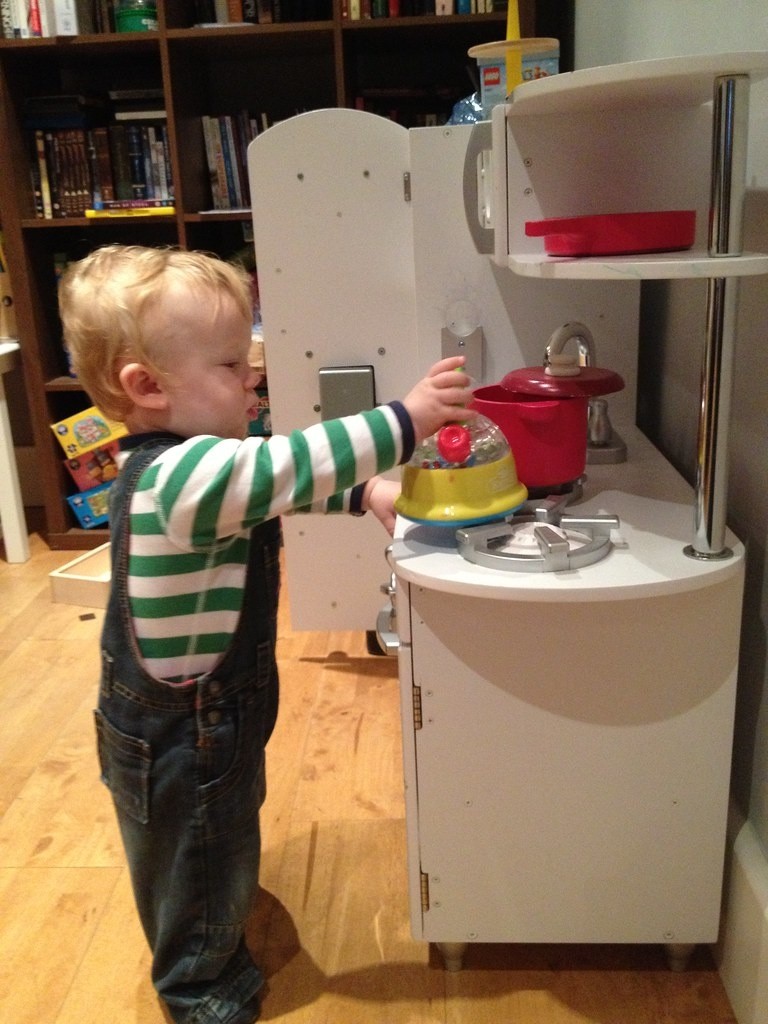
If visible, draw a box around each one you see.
[0,0,110,38]
[27,89,176,219]
[355,97,448,128]
[341,0,493,20]
[201,112,301,211]
[213,0,281,24]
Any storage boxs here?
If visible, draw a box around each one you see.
[248,389,271,437]
[50,406,130,530]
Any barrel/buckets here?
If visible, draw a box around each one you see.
[468,34,560,124]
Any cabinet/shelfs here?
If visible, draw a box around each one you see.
[376,49,768,971]
[1,1,575,550]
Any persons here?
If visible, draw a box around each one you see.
[59,244,479,1024]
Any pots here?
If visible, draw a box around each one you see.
[468,363,625,498]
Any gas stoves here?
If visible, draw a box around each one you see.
[390,423,745,602]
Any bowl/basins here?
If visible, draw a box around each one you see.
[114,7,158,32]
